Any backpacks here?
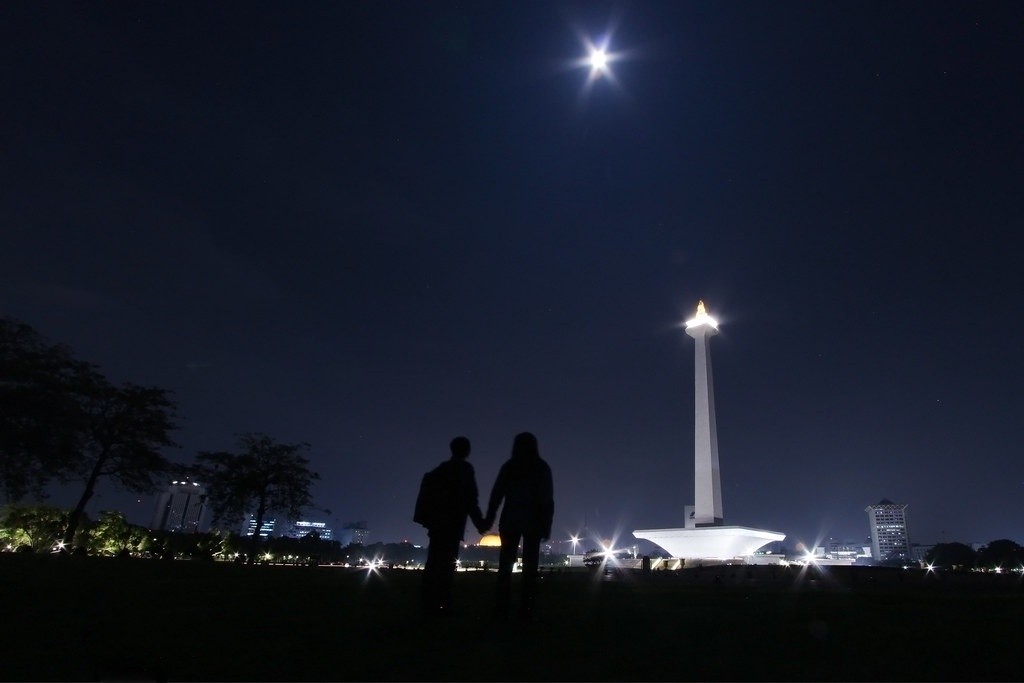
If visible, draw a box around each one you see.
[413,472,436,529]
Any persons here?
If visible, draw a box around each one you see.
[411,436,484,573]
[485,432,554,576]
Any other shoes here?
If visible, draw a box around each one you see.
[520,607,535,623]
[491,607,513,624]
